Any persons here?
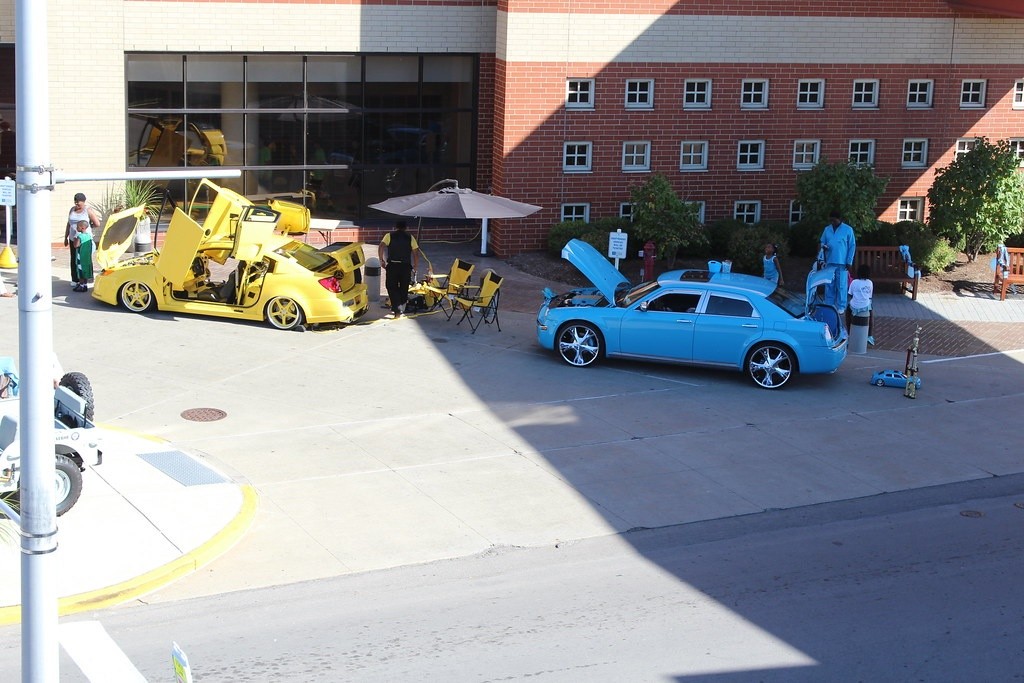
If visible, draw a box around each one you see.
[259,139,272,189]
[309,138,329,204]
[817,208,856,270]
[72,221,92,292]
[378,221,419,318]
[845,264,875,346]
[64,193,101,287]
[347,137,362,212]
[762,244,784,287]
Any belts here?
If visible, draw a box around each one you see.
[389,259,404,263]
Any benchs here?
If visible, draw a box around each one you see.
[993,246,1024,300]
[849,246,920,301]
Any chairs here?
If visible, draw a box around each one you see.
[423,258,475,321]
[454,269,504,335]
[200,261,245,300]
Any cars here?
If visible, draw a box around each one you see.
[870,369,921,390]
[339,126,453,202]
[536,239,851,390]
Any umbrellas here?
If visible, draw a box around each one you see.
[367,179,543,240]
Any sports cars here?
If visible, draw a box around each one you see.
[90,178,370,332]
[124,103,228,221]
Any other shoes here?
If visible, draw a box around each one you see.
[867,336,875,346]
[72,284,88,292]
[70,281,78,286]
[395,310,403,318]
[837,309,844,314]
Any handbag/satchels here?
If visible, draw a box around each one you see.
[847,271,853,286]
